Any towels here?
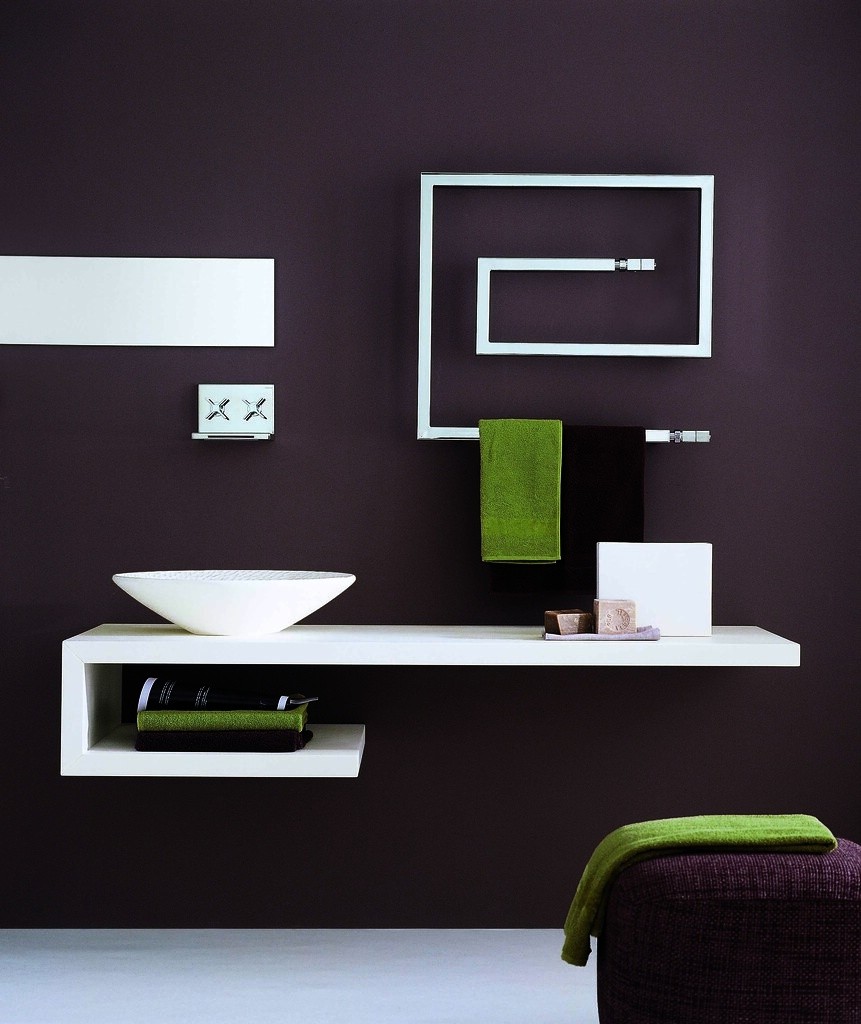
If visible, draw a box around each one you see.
[559,815,840,967]
[482,417,563,561]
[134,711,313,753]
[562,413,646,564]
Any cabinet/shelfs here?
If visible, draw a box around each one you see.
[57,623,803,779]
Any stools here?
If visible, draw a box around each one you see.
[598,837,861,1024]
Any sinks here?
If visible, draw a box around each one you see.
[112,569,358,635]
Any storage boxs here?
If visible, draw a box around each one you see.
[597,542,715,639]
